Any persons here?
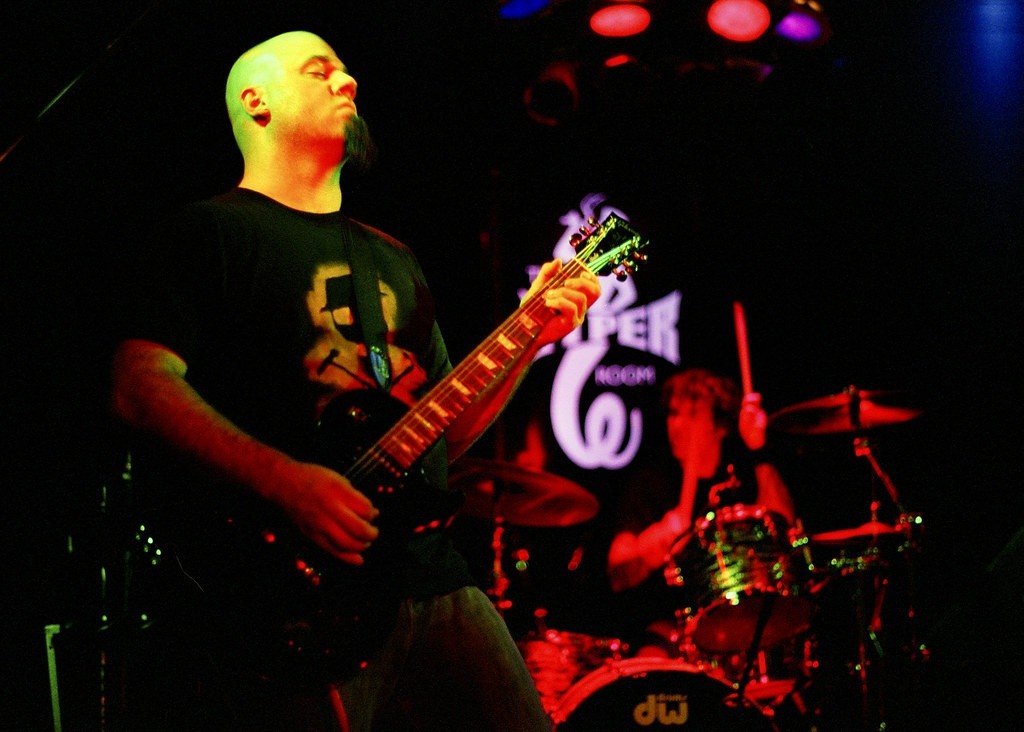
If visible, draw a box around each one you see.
[603,372,810,659]
[112,29,599,732]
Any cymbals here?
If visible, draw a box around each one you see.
[806,498,904,548]
[445,454,602,530]
[768,383,923,435]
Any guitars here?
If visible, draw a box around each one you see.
[197,209,652,696]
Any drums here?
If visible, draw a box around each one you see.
[674,503,818,657]
[547,652,780,732]
